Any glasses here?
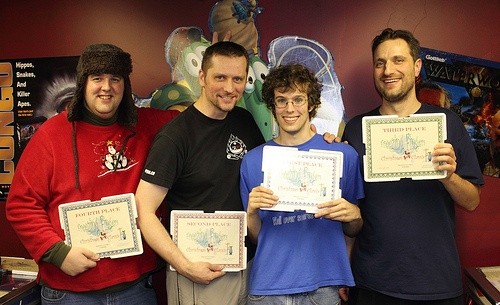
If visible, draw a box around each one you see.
[272,97,308,108]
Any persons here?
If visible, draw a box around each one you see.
[134,41,266,305]
[240,63,364,305]
[5,43,182,305]
[310,27,486,305]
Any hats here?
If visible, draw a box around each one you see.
[67,44,137,127]
